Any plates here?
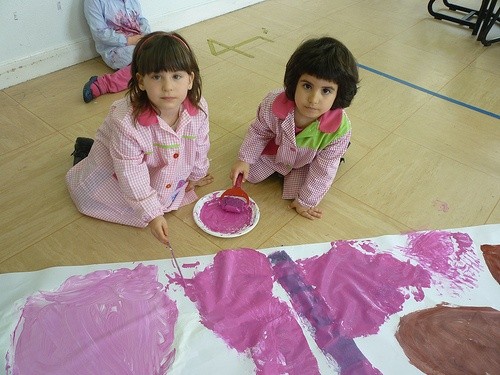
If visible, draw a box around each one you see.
[192,190,261,239]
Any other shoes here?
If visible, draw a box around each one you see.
[83,76,101,103]
[70,137,94,166]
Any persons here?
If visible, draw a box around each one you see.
[65,31,214,244]
[82,0,152,103]
[229,37,359,220]
